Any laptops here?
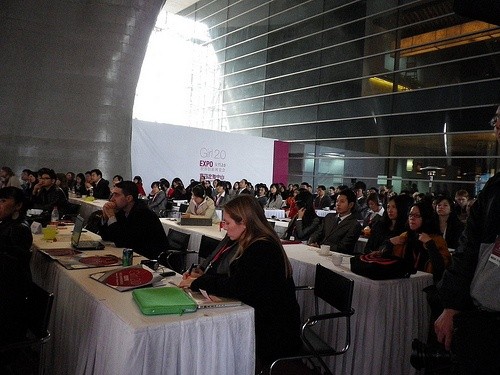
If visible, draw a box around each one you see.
[71,214,105,250]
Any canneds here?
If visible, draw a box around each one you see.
[122,249,133,267]
[220,221,225,231]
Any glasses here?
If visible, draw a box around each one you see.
[39,177,50,180]
[408,212,422,218]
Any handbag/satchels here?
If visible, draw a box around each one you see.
[469,235,500,315]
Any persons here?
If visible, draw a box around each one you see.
[416,101,500,375]
[178,192,304,375]
[0,164,490,367]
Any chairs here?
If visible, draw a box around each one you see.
[158,228,191,274]
[60,203,83,222]
[269,264,355,375]
[179,204,189,213]
[167,235,221,275]
[0,279,54,375]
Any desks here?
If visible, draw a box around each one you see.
[29,191,435,375]
